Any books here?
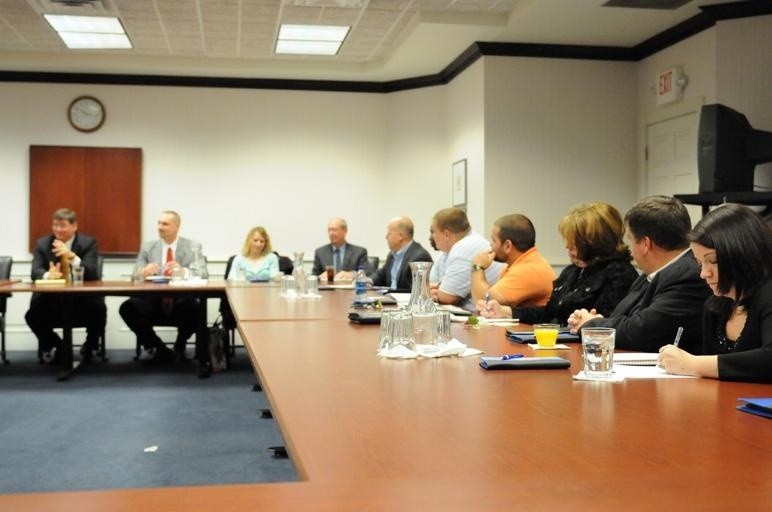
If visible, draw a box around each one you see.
[736,396,771,419]
[505,328,581,343]
[347,295,397,324]
[479,356,571,370]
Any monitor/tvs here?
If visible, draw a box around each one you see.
[696,102,772,193]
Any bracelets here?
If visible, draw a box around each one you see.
[67,253,76,261]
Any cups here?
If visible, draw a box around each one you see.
[280,276,289,289]
[131,265,144,284]
[171,269,184,284]
[355,266,366,299]
[580,326,616,375]
[73,267,85,283]
[378,310,403,350]
[534,322,561,346]
[326,265,335,283]
[286,278,297,292]
[388,316,414,352]
[306,276,317,292]
[435,309,450,347]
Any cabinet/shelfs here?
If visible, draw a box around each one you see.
[672,191,772,220]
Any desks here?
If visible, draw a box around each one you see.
[226,277,468,319]
[1,277,225,368]
[1,319,772,510]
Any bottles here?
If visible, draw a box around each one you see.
[291,252,307,290]
[403,261,438,345]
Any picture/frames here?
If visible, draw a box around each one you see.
[452,160,467,206]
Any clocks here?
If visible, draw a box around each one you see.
[69,96,104,131]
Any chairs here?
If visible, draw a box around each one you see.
[133,298,207,361]
[0,256,12,367]
[224,256,293,358]
[38,256,108,362]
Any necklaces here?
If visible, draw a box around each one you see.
[712,316,749,353]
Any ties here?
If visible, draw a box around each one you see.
[336,251,342,272]
[165,249,172,277]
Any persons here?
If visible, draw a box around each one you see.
[119,211,209,371]
[477,202,639,329]
[24,207,107,366]
[567,194,713,352]
[657,203,772,382]
[364,207,554,326]
[227,227,281,284]
[311,219,368,282]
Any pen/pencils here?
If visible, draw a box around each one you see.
[484,291,489,317]
[673,327,684,347]
[503,354,524,360]
